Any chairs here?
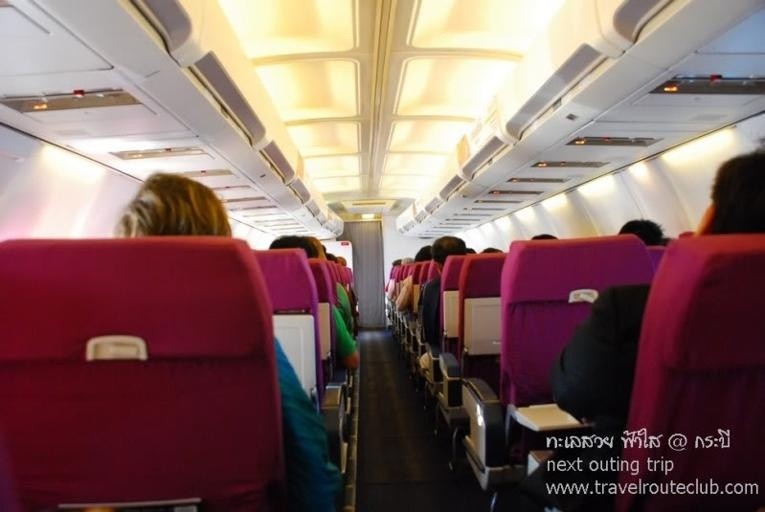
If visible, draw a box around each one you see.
[426,259,445,394]
[2,239,287,511]
[389,262,430,359]
[612,233,765,512]
[463,236,655,512]
[255,249,347,473]
[646,245,665,277]
[309,255,348,385]
[440,251,507,428]
[326,261,352,291]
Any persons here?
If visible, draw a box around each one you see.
[422,235,467,344]
[114,171,345,512]
[491,149,764,512]
[395,246,439,311]
[621,219,663,246]
[268,236,360,371]
[303,237,353,336]
